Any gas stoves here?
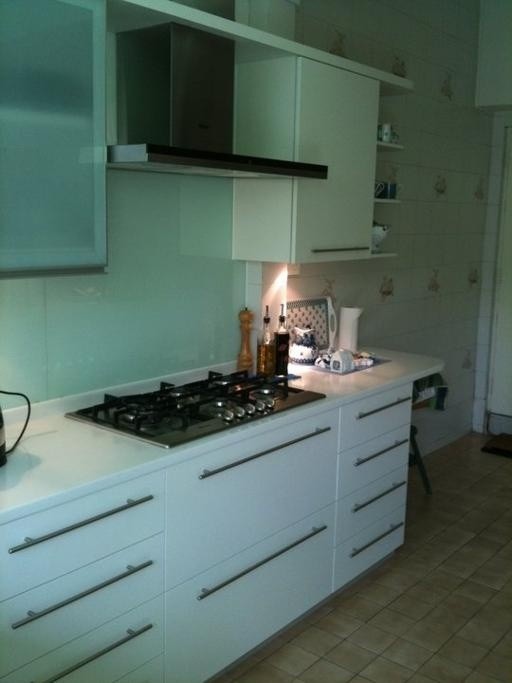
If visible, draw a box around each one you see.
[64,365,327,450]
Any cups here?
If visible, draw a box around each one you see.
[378,121,393,143]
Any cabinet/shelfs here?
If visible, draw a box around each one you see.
[0,386,415,683]
[231,55,414,265]
[1,0,109,278]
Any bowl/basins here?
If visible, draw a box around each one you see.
[370,223,392,254]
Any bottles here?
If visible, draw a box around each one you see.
[255,315,279,375]
[273,315,293,374]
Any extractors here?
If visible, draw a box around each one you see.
[106,0,330,181]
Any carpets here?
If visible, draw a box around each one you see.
[481,432,512,458]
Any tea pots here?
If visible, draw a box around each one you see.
[289,324,320,364]
